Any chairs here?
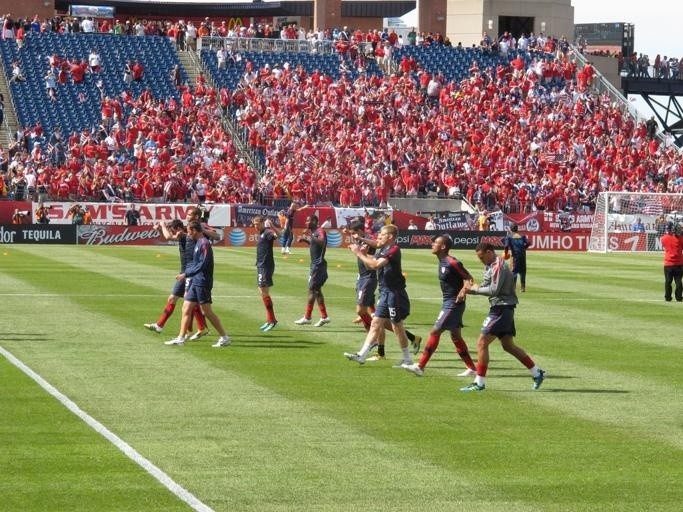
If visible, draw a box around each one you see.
[389,34,572,121]
[1,23,200,158]
[203,44,384,158]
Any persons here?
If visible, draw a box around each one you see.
[558,212,575,232]
[631,217,645,232]
[461,28,683,215]
[1,11,240,204]
[385,213,392,225]
[143,216,209,336]
[504,221,531,292]
[34,202,49,224]
[11,208,24,224]
[425,216,437,231]
[277,202,310,255]
[407,219,418,230]
[401,234,477,377]
[237,15,462,209]
[654,216,683,302]
[343,222,378,336]
[459,241,546,391]
[175,206,221,340]
[253,215,279,332]
[479,210,491,232]
[343,223,414,369]
[124,203,142,226]
[69,203,93,224]
[350,216,377,323]
[363,211,373,234]
[294,214,331,327]
[164,221,232,347]
[365,233,422,361]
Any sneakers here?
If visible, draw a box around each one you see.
[144,324,162,334]
[263,321,278,331]
[344,352,365,365]
[411,336,421,355]
[352,316,363,323]
[458,369,477,376]
[165,340,184,346]
[213,338,231,347]
[295,317,312,325]
[315,317,330,327]
[402,363,424,376]
[190,329,208,339]
[367,352,386,360]
[460,384,485,391]
[393,359,413,369]
[533,370,547,388]
[261,322,269,330]
